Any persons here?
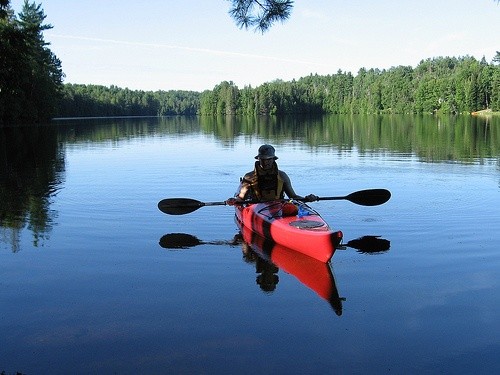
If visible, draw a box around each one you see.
[230,234,280,292]
[227,144,320,204]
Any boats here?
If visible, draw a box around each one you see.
[234,183,343,263]
[234,213,344,318]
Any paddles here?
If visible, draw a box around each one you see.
[156,188,392,216]
[158,231,392,256]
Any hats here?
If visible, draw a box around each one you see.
[255,145,279,161]
